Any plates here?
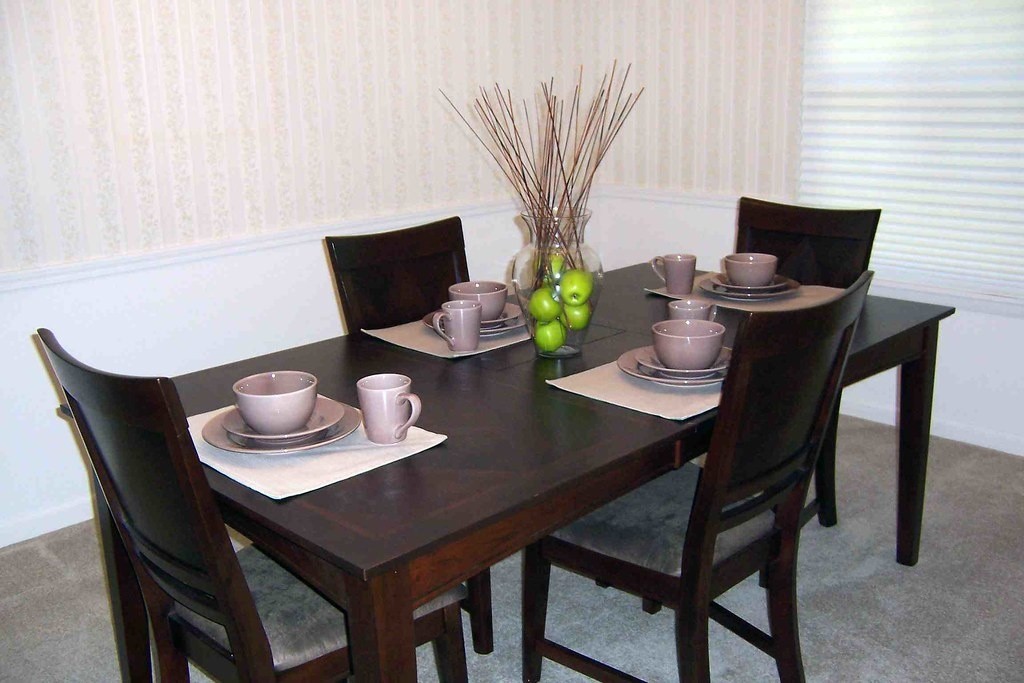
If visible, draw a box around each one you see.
[711,273,788,293]
[618,349,728,387]
[635,346,732,379]
[202,403,362,455]
[423,312,526,337]
[699,278,801,301]
[222,397,345,444]
[433,303,522,330]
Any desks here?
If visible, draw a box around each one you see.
[168,263,956,683]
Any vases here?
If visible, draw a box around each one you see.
[511,207,604,360]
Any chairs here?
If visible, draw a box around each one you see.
[519,269,873,683]
[37,326,470,683]
[736,197,881,588]
[324,216,611,655]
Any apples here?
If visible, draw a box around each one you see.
[527,253,593,351]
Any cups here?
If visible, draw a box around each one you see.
[356,374,421,445]
[668,300,717,322]
[232,371,318,434]
[433,300,482,353]
[448,281,508,321]
[725,253,777,287]
[652,319,726,369]
[652,253,696,295]
